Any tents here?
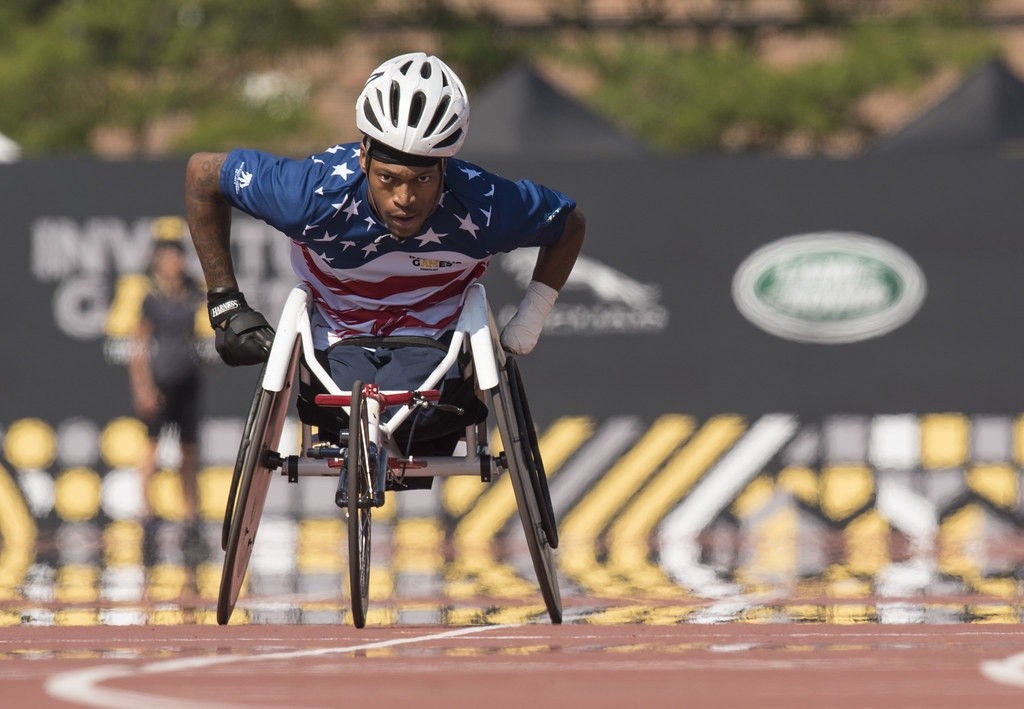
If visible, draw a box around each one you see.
[432,62,641,155]
[892,58,1024,143]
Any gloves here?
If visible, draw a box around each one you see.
[501,280,559,355]
[206,287,276,367]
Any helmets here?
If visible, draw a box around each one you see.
[354,51,470,157]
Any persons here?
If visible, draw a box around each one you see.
[185,51,587,458]
[124,240,213,468]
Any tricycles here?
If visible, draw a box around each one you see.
[216,280,565,629]
